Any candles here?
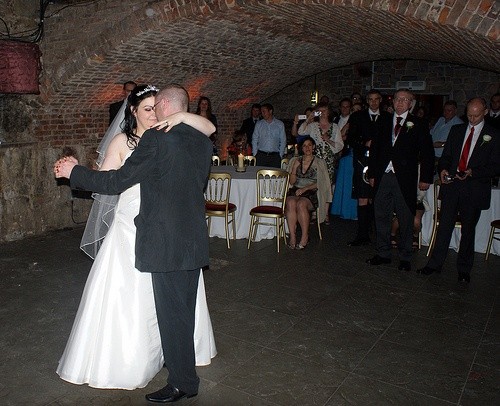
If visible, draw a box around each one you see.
[238,153,243,168]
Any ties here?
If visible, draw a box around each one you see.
[370,114,376,122]
[459,126,475,171]
[394,117,403,137]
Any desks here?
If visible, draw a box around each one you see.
[421,184,500,256]
[204,165,289,242]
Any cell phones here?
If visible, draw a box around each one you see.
[313,111,323,117]
[297,115,307,120]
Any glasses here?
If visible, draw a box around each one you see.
[395,97,412,102]
[152,102,161,111]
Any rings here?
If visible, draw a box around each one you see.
[299,192,302,196]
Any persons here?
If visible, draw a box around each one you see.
[417,96,494,283]
[285,137,332,249]
[54,84,213,403]
[251,103,287,168]
[367,89,435,271]
[292,93,423,166]
[109,81,138,123]
[194,97,219,166]
[298,102,344,225]
[389,164,427,249]
[241,103,262,131]
[228,129,251,164]
[331,102,363,221]
[348,90,388,247]
[484,92,500,188]
[431,100,465,172]
[52,84,217,391]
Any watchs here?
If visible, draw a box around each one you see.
[441,142,445,147]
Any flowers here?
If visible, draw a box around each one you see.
[481,135,492,146]
[406,122,414,132]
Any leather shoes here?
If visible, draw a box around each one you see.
[416,266,434,275]
[348,238,367,248]
[145,383,197,403]
[454,272,471,282]
[400,260,410,271]
[366,254,392,264]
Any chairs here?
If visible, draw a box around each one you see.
[426,179,461,256]
[231,156,256,166]
[204,172,237,249]
[485,220,500,260]
[248,169,289,252]
[310,207,322,240]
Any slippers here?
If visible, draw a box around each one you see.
[296,236,310,250]
[288,238,296,250]
